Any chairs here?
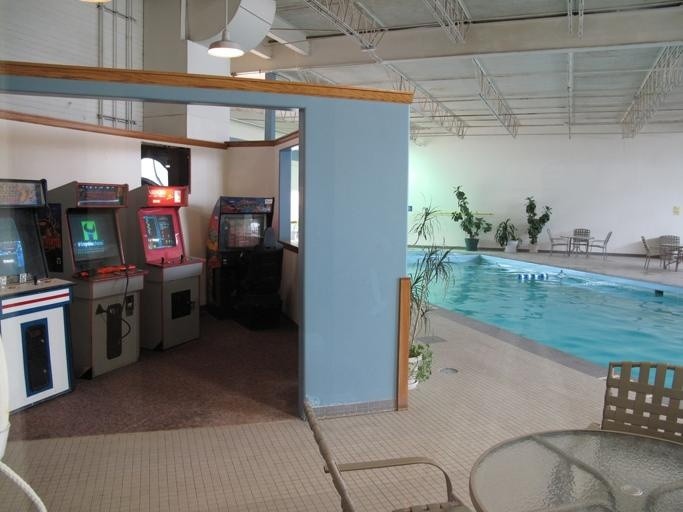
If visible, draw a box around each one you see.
[601,359,681,443]
[639,233,683,275]
[546,227,613,262]
[297,395,472,511]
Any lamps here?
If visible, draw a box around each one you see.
[207,0,247,62]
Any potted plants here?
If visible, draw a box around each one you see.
[404,244,458,391]
[448,184,553,255]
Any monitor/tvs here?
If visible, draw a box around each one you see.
[219,214,267,251]
[0,208,46,280]
[136,208,184,259]
[65,208,125,272]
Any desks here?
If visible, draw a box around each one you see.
[468,429,681,510]
